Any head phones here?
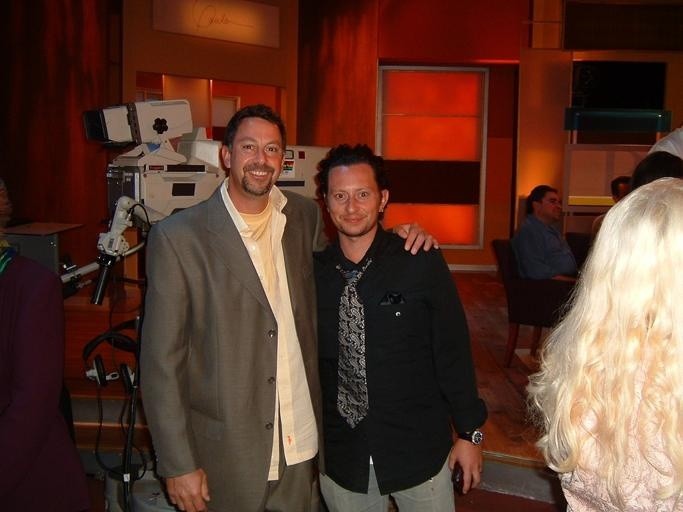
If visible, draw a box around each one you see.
[82,330,139,392]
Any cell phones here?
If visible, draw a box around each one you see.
[451,458,472,493]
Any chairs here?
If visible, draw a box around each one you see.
[567,232,591,267]
[493,240,576,368]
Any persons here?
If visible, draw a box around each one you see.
[609,173,634,203]
[0,178,88,512]
[522,176,681,512]
[647,125,681,162]
[309,143,486,512]
[507,182,579,285]
[138,101,439,511]
[633,151,681,191]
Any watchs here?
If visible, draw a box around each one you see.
[457,430,482,446]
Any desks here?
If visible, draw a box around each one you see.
[3,222,84,299]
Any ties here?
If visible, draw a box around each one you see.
[337,258,374,430]
